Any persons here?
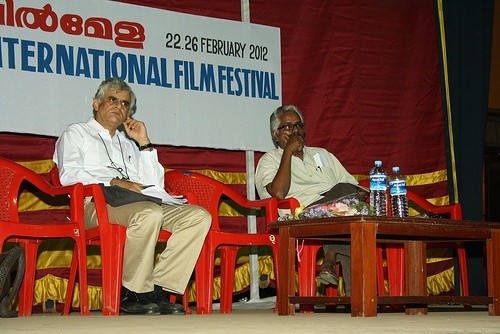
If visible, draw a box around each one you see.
[53,79,212,315]
[254,105,360,296]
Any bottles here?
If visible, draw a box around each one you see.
[389,166,409,218]
[370,160,387,217]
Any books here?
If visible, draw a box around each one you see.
[303,182,370,209]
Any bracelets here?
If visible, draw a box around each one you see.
[140,143,152,152]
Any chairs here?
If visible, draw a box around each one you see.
[162,168,288,317]
[50,163,212,318]
[256,183,397,315]
[357,179,472,309]
[0,158,91,316]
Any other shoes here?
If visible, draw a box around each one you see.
[317,261,340,287]
[119,285,161,315]
[140,285,184,315]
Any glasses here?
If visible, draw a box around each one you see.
[107,162,130,180]
[277,123,306,132]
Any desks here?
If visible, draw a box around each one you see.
[266,213,500,317]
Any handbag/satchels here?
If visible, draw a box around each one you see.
[91,182,163,207]
